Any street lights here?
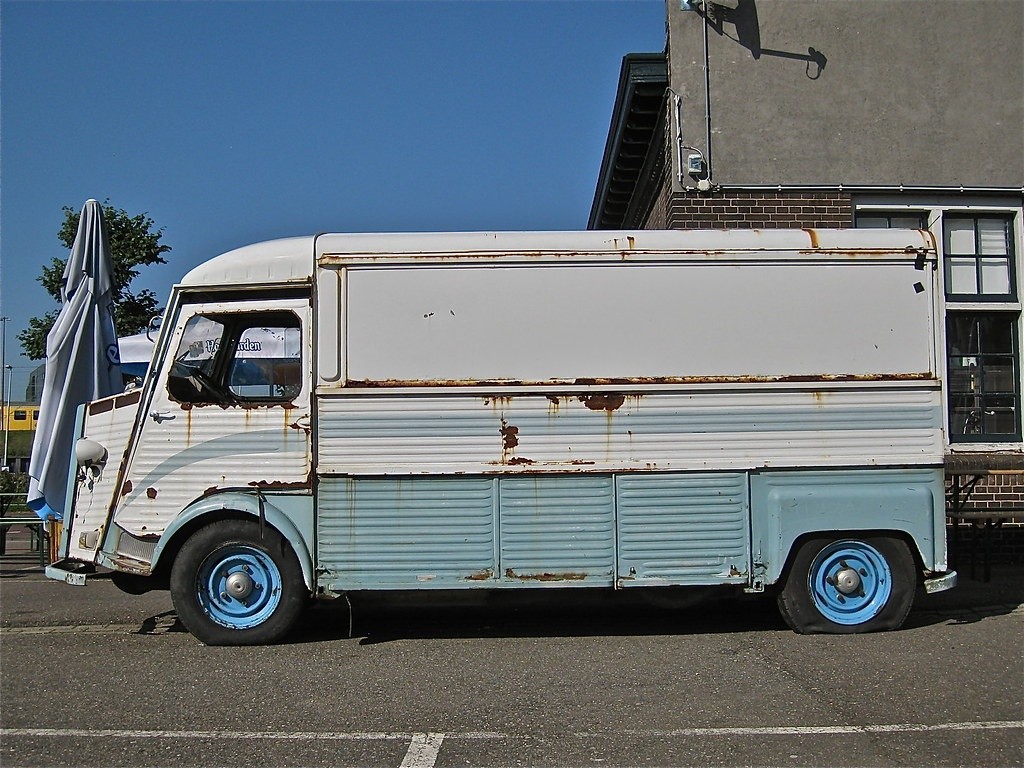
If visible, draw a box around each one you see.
[1,364,14,471]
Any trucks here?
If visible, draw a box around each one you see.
[35,227,960,650]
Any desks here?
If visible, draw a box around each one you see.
[0,493,48,567]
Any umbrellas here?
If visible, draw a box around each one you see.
[26,199,125,523]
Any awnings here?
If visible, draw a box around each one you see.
[116,319,301,397]
